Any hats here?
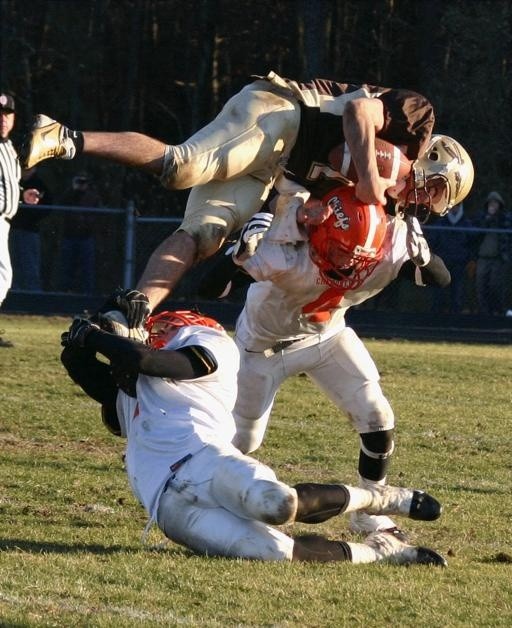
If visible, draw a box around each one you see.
[0,92,16,113]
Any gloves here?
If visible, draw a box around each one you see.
[61,317,95,345]
[225,211,273,265]
[405,213,430,267]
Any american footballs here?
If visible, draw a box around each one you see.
[330,133,411,181]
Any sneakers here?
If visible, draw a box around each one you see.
[113,283,152,327]
[99,312,149,350]
[350,510,410,545]
[365,530,446,566]
[21,114,65,170]
[0,329,13,346]
[368,486,439,522]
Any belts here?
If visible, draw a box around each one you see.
[256,338,302,356]
[1,215,12,224]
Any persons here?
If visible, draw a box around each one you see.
[0,94,40,349]
[224,185,457,542]
[470,192,512,318]
[52,176,105,294]
[17,69,482,347]
[58,286,447,571]
[13,159,51,291]
[428,201,472,314]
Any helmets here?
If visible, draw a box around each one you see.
[144,309,224,350]
[308,185,388,289]
[395,135,475,223]
